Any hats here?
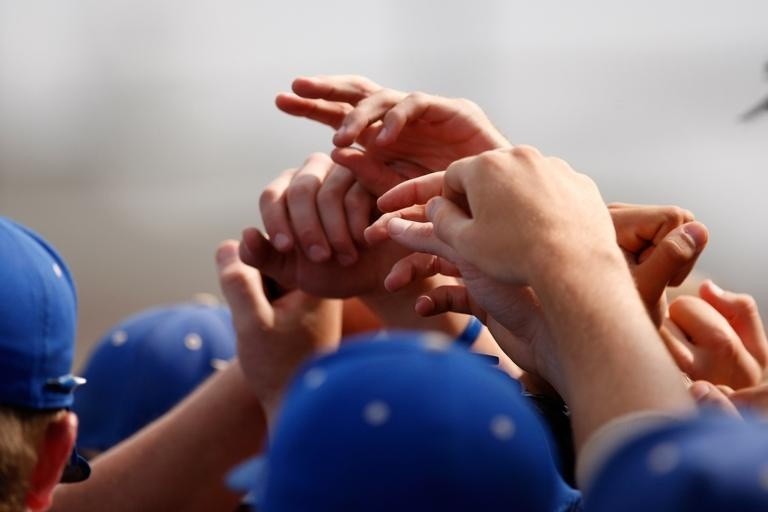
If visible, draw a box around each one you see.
[250,330,585,510]
[0,216,88,411]
[75,304,241,452]
[580,413,768,512]
